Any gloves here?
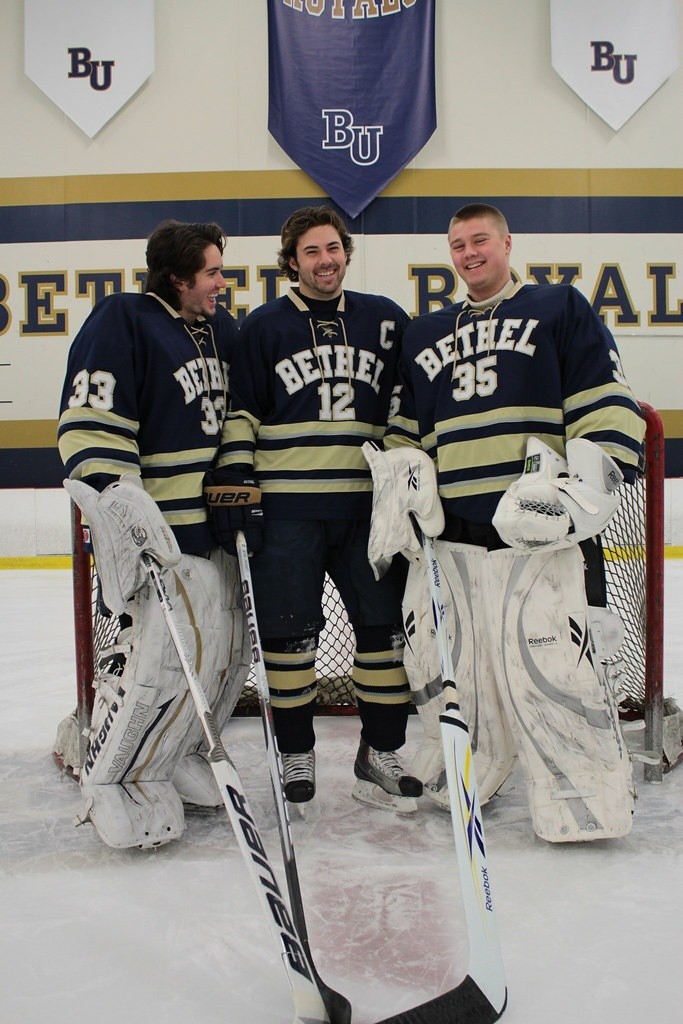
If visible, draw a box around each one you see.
[201,462,263,559]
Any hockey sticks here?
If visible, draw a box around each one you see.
[354,429,512,1024]
[226,508,355,1024]
[136,549,359,1024]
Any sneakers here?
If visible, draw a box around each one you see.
[279,748,315,819]
[350,734,423,818]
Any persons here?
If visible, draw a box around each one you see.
[57,221,253,849]
[203,206,423,803]
[380,204,647,842]
